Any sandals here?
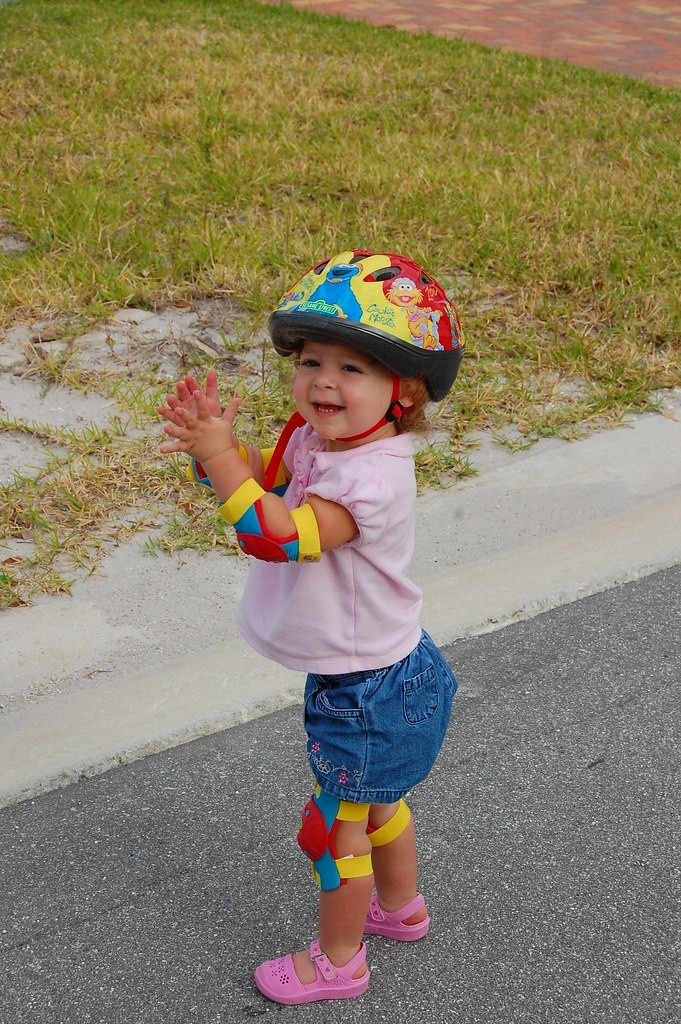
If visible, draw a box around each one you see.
[362,891,431,943]
[255,941,372,1004]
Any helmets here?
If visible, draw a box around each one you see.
[263,250,466,404]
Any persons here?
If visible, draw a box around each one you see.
[156,249,459,1003]
